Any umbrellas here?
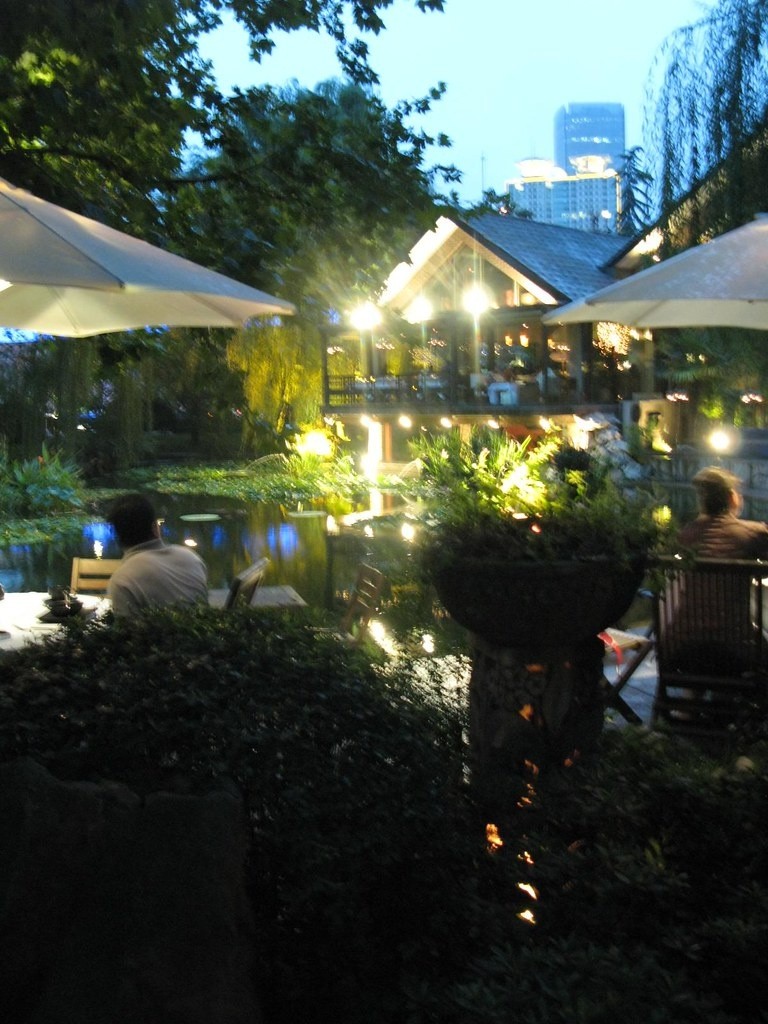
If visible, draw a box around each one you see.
[541,216,768,331]
[0,180,297,338]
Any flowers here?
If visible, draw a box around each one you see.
[574,410,645,497]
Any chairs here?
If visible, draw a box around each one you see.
[67,558,121,617]
[336,562,385,650]
[223,558,269,610]
[652,557,768,746]
[518,384,539,405]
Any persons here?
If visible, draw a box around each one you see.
[109,495,208,620]
[363,346,642,406]
[664,467,768,721]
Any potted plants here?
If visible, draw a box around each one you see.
[391,499,678,668]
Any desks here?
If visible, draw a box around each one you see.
[0,589,112,651]
[350,380,503,407]
[207,586,307,615]
